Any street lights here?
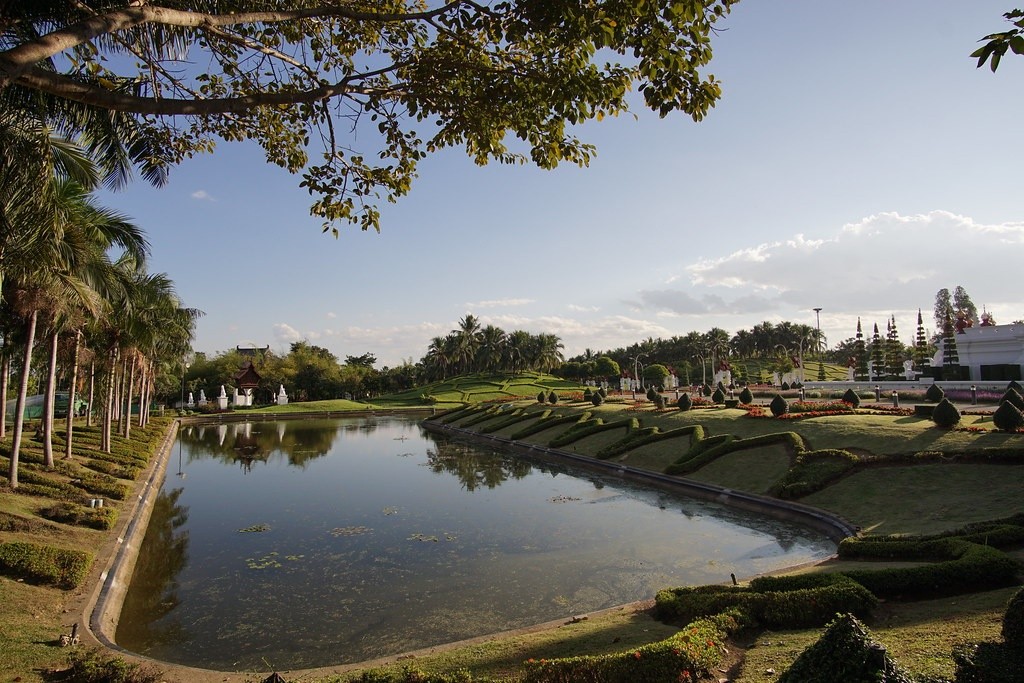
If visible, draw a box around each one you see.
[629,353,649,388]
[774,344,793,357]
[791,335,809,382]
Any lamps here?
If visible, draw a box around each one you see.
[802,385,805,390]
[736,383,739,387]
[875,385,880,391]
[730,389,734,394]
[971,385,976,391]
[697,384,703,389]
[675,388,679,393]
[892,390,899,397]
[798,390,803,395]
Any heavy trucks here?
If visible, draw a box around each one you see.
[55,391,89,417]
